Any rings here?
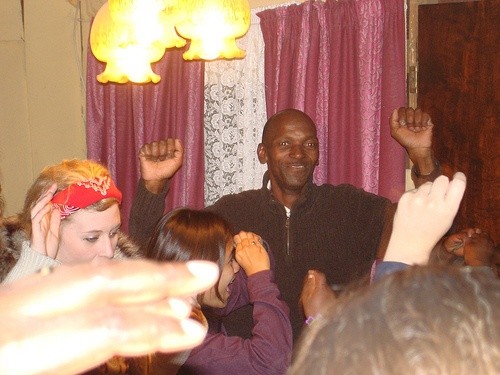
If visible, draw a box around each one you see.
[252,241,257,244]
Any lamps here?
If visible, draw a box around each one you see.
[169,1,251,61]
[89,0,185,84]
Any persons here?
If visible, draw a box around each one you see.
[146,208,292,375]
[0,158,209,375]
[0,258,219,375]
[125,105,442,343]
[431,228,500,274]
[285,172,500,375]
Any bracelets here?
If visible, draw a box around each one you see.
[408,155,439,179]
